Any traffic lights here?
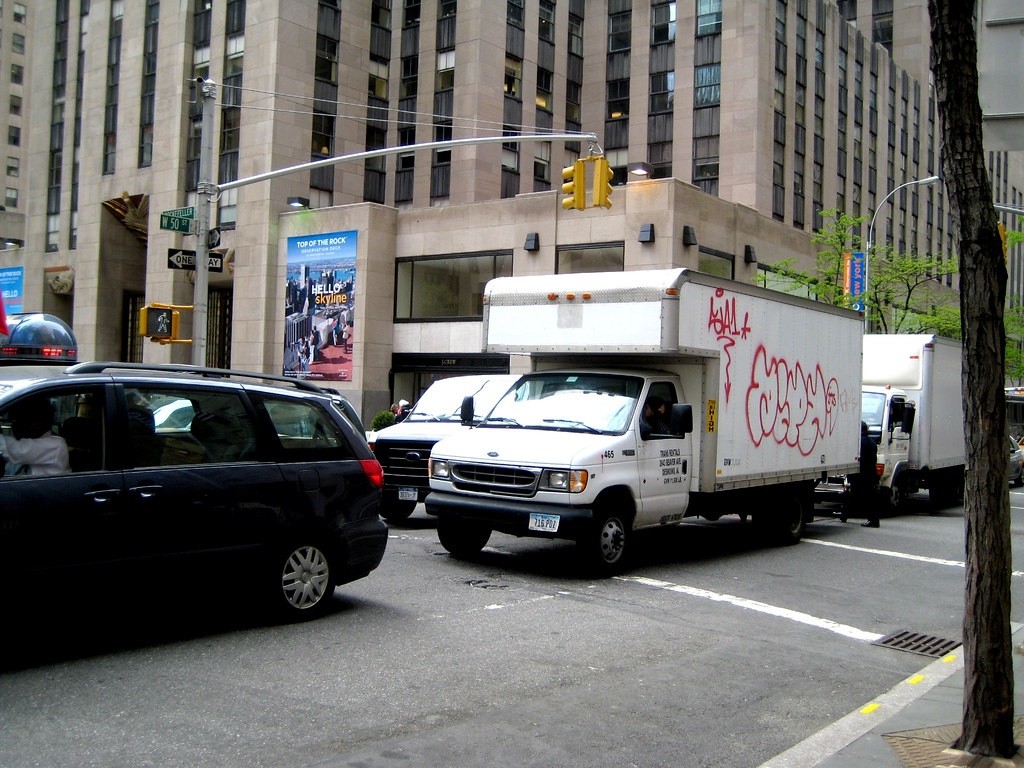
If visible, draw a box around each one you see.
[141,305,178,340]
[560,161,587,212]
[593,157,614,209]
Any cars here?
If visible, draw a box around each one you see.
[1002,387,1024,489]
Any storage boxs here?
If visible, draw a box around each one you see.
[161,436,205,465]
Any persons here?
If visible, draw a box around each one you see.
[0,396,72,477]
[390,399,412,424]
[832,421,880,528]
[642,395,673,435]
[297,318,352,376]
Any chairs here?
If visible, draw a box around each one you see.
[61,417,95,471]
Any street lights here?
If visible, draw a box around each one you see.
[863,174,940,335]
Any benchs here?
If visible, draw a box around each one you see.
[181,437,257,463]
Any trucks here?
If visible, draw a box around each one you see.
[423,266,866,578]
[814,333,966,519]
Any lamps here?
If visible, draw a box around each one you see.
[286,194,315,211]
[4,238,24,248]
[627,163,655,180]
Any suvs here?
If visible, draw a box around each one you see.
[375,371,529,525]
[0,363,388,646]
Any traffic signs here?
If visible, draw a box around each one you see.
[168,249,224,274]
[207,226,219,248]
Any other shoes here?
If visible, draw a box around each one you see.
[860,520,871,526]
[833,512,848,523]
